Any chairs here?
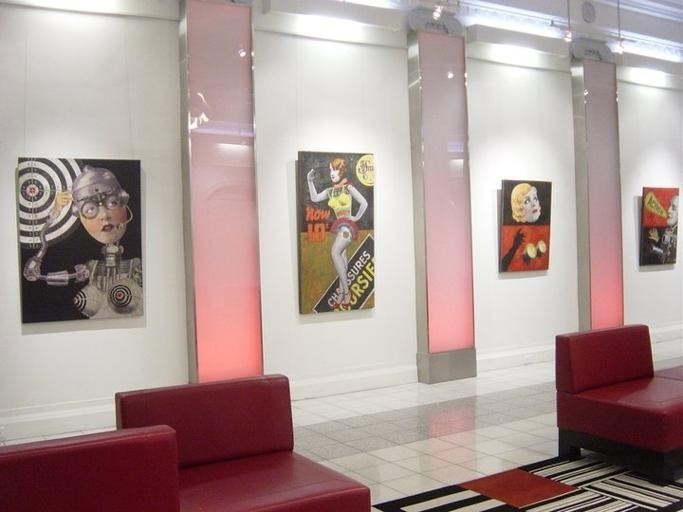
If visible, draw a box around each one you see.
[0,424,179,511]
[114,372,371,512]
[546,324,683,486]
[654,362,681,383]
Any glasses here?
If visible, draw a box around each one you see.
[74,188,121,219]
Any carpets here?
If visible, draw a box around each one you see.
[374,452,683,509]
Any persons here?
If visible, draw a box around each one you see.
[510,183,542,223]
[501,228,526,271]
[23,165,142,318]
[307,159,369,308]
[648,193,679,226]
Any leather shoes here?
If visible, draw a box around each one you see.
[343,291,352,308]
[333,292,344,308]
